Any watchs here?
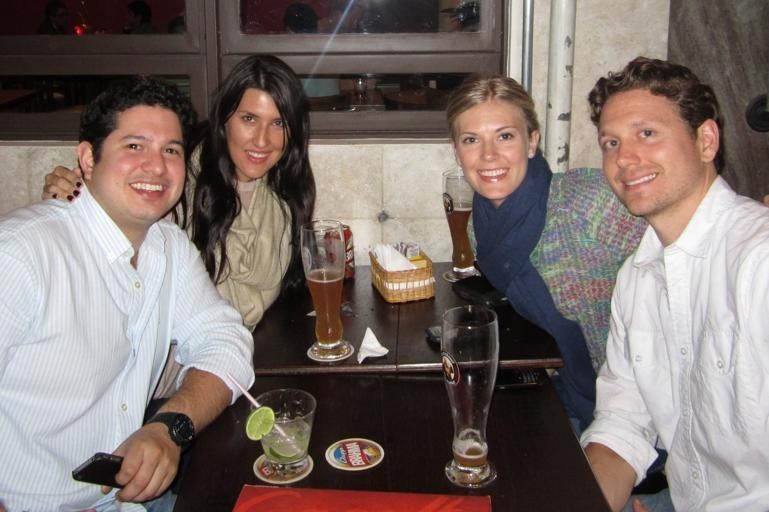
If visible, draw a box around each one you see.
[146,411,197,454]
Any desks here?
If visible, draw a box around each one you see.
[252,260,564,375]
[171,366,613,512]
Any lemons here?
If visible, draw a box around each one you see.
[245,406,274,441]
[268,447,300,460]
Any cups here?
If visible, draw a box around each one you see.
[439,304,499,490]
[299,217,355,360]
[442,170,476,272]
[253,389,315,463]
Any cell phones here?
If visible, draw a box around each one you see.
[426,326,442,343]
[481,362,547,390]
[72,452,125,488]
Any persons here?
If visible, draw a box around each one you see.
[39,54,320,396]
[576,56,769,512]
[446,73,684,512]
[0,70,256,512]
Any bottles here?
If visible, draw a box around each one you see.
[355,72,369,104]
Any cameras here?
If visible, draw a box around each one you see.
[453,276,508,309]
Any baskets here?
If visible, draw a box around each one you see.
[369,248,436,303]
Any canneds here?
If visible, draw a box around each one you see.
[323,225,353,278]
